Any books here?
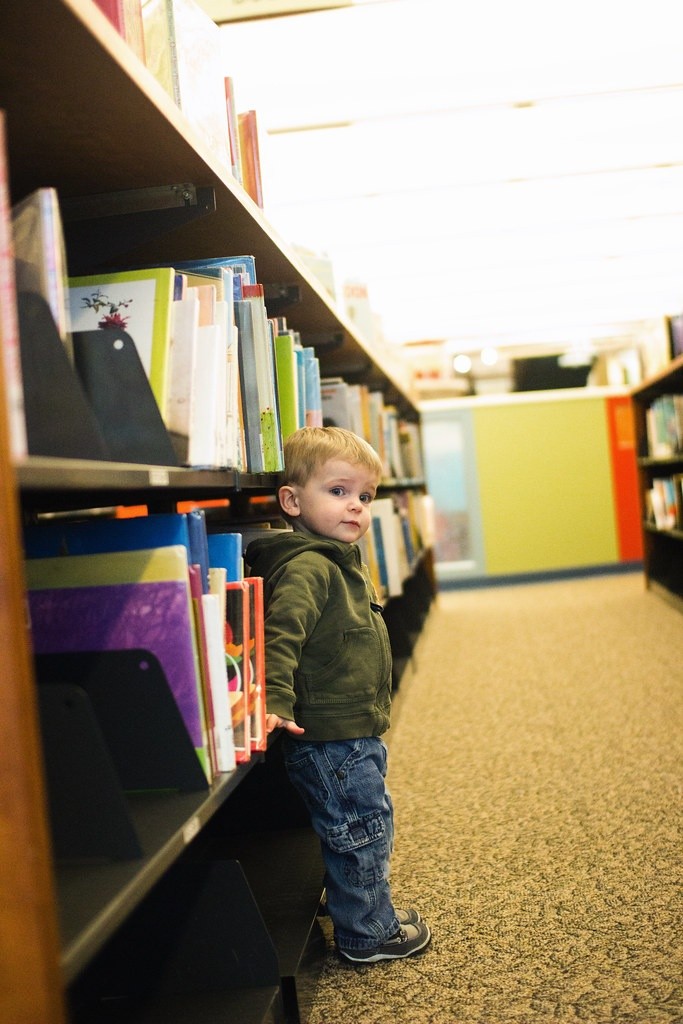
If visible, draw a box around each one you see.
[643,392,682,535]
[15,185,429,789]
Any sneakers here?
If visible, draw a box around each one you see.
[336,905,430,966]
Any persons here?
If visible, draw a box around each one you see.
[246,428,429,965]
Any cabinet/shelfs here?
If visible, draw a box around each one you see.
[416,384,647,585]
[626,312,683,600]
[0,0,440,1024]
